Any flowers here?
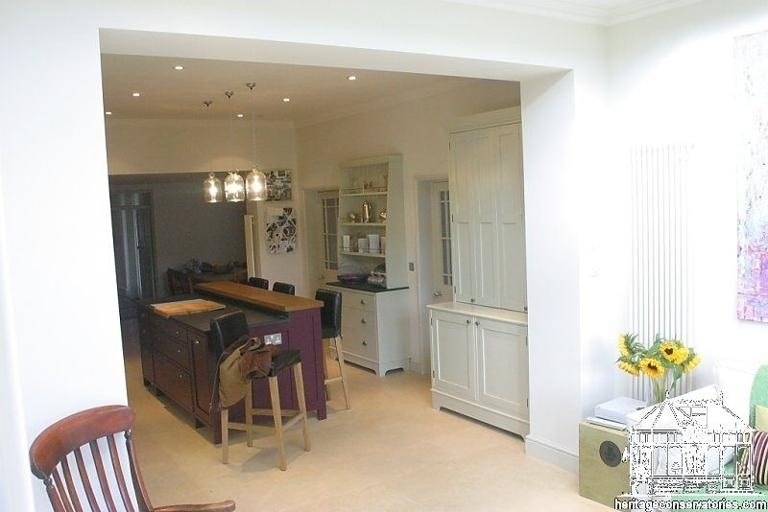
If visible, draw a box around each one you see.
[617,332,700,405]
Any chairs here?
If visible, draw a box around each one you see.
[28,406,233,512]
[674,366,768,511]
[210,309,313,471]
[247,278,350,411]
[168,267,246,295]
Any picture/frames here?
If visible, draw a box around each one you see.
[260,166,300,256]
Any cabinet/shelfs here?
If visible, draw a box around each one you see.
[426,301,529,441]
[445,122,525,315]
[336,153,408,289]
[137,293,294,449]
[325,283,407,376]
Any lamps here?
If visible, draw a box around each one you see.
[201,81,269,205]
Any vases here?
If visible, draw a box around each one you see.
[649,371,669,406]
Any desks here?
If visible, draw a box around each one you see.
[186,262,242,282]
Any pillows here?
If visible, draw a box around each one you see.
[737,405,768,484]
